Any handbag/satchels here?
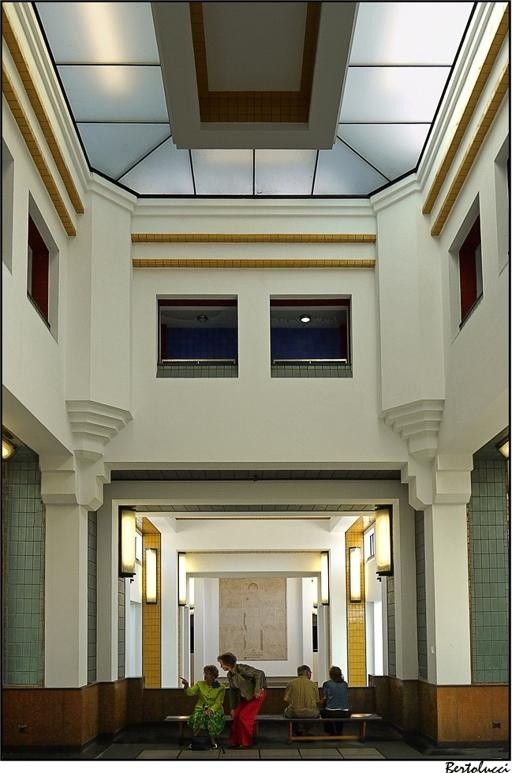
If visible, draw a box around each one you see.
[191,727,209,750]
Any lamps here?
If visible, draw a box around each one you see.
[321,507,391,607]
[119,507,187,607]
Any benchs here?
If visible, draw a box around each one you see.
[164,712,384,745]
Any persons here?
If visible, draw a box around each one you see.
[282,665,352,741]
[179,652,267,750]
